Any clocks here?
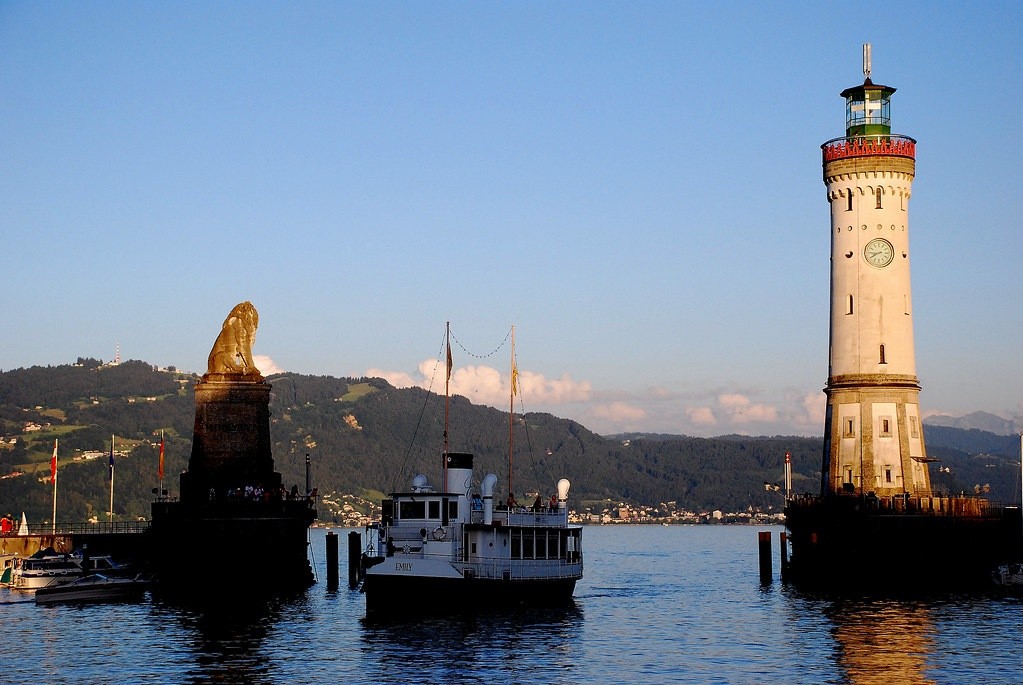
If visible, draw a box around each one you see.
[864,239,893,268]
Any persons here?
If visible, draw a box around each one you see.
[209,482,299,503]
[506,493,520,509]
[549,495,559,513]
[496,500,506,510]
[531,496,542,512]
[308,486,318,509]
[1,513,15,537]
[386,536,402,557]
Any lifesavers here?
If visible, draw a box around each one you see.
[433,525,447,540]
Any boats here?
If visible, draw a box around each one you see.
[360,321,585,607]
[7,543,165,605]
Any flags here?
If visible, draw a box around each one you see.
[109,442,115,480]
[510,368,519,396]
[49,443,57,484]
[158,433,165,479]
[448,345,452,380]
